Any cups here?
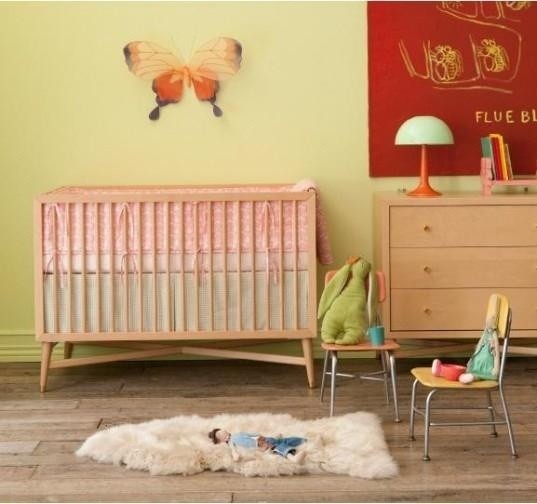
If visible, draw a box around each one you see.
[368,325,385,346]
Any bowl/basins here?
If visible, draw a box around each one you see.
[438,364,466,381]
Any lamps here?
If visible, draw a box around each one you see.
[395,114,456,198]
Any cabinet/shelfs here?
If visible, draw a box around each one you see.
[374,191,537,374]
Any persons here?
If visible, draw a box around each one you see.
[208,428,324,464]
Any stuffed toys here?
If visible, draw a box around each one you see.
[317,255,379,345]
[432,296,500,384]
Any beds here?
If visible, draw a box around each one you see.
[32,184,333,392]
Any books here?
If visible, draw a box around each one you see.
[480,133,514,181]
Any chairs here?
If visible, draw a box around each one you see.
[318,269,403,424]
[408,293,514,461]
